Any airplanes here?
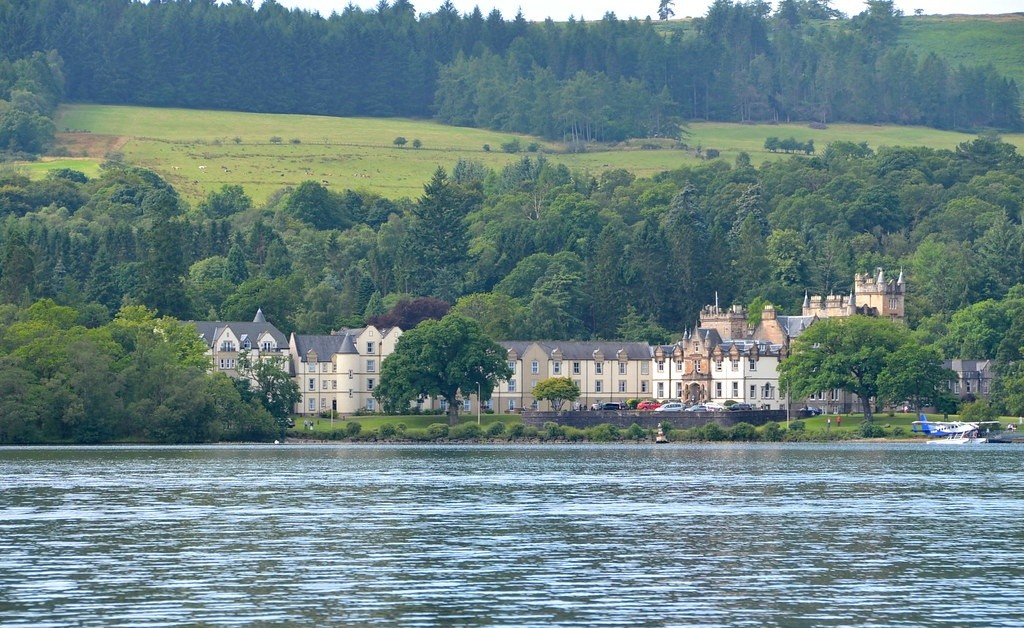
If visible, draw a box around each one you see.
[911,412,998,445]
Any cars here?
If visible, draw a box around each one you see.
[637,400,661,410]
[795,406,822,418]
[727,402,752,411]
[591,403,601,410]
[706,402,724,412]
[685,404,708,413]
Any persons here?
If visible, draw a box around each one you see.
[904,405,909,413]
[510,394,696,411]
[836,416,841,426]
[658,422,662,428]
[972,423,1017,438]
[826,418,832,428]
[304,418,314,430]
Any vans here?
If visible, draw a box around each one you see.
[277,417,296,428]
[602,401,622,410]
[655,402,683,412]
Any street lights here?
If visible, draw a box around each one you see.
[474,381,480,426]
